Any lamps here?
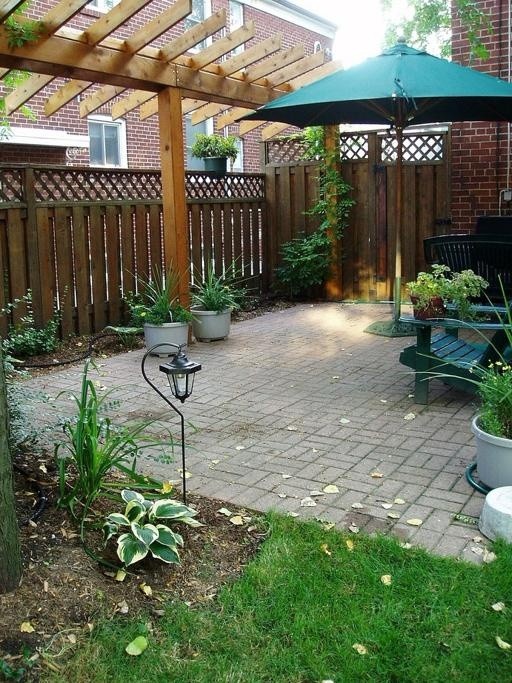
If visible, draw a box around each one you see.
[141,341,203,504]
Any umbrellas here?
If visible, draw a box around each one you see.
[233,34,512,323]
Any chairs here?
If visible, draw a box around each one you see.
[424,235,511,300]
[398,302,511,404]
[474,217,511,251]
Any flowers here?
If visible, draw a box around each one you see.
[403,273,511,438]
[190,255,260,313]
[124,264,192,326]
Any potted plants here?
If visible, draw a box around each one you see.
[192,135,238,172]
[407,264,489,320]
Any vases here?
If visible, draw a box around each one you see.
[471,411,512,488]
[143,322,189,358]
[190,304,234,343]
